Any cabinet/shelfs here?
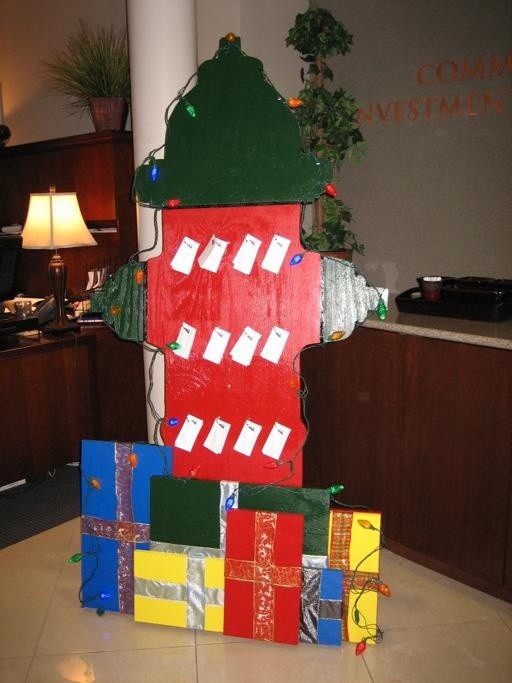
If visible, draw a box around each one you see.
[1,129,140,335]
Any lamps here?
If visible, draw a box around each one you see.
[16,182,100,335]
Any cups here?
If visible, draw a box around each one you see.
[415,275,445,303]
[14,300,32,318]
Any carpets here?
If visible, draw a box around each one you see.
[1,464,80,549]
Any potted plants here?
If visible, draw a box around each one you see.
[285,1,369,260]
[40,16,132,130]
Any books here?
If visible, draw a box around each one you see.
[77,316,104,323]
